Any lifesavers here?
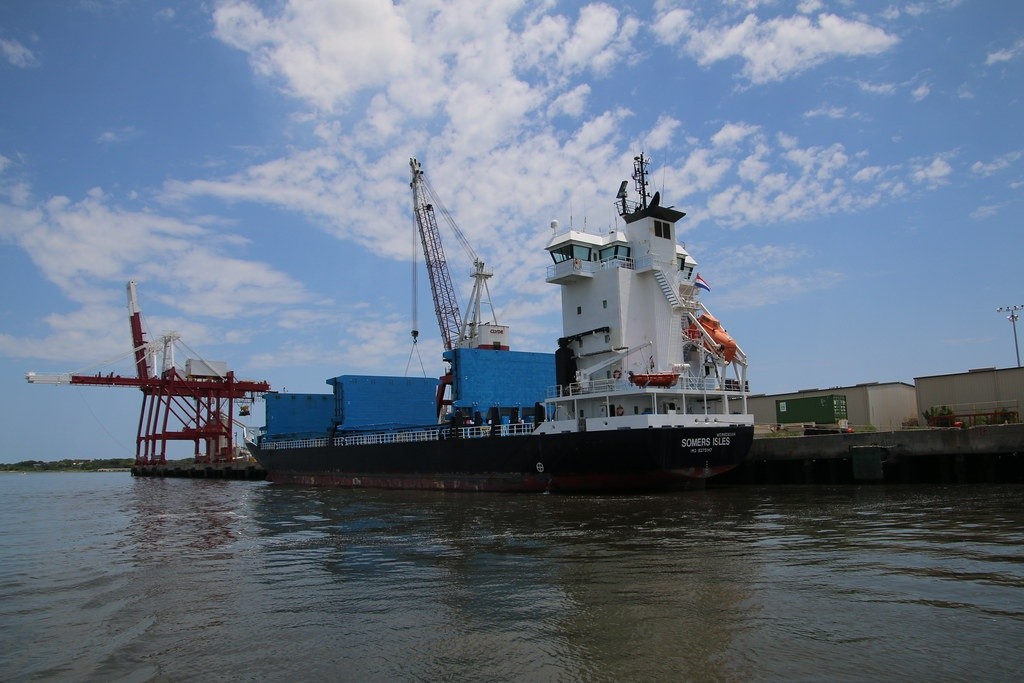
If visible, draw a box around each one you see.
[617,407,624,415]
[612,370,622,379]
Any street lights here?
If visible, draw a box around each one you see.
[996,305,1023,369]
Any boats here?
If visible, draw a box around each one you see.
[243,148,756,494]
[627,371,681,386]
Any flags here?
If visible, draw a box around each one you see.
[695,275,711,292]
